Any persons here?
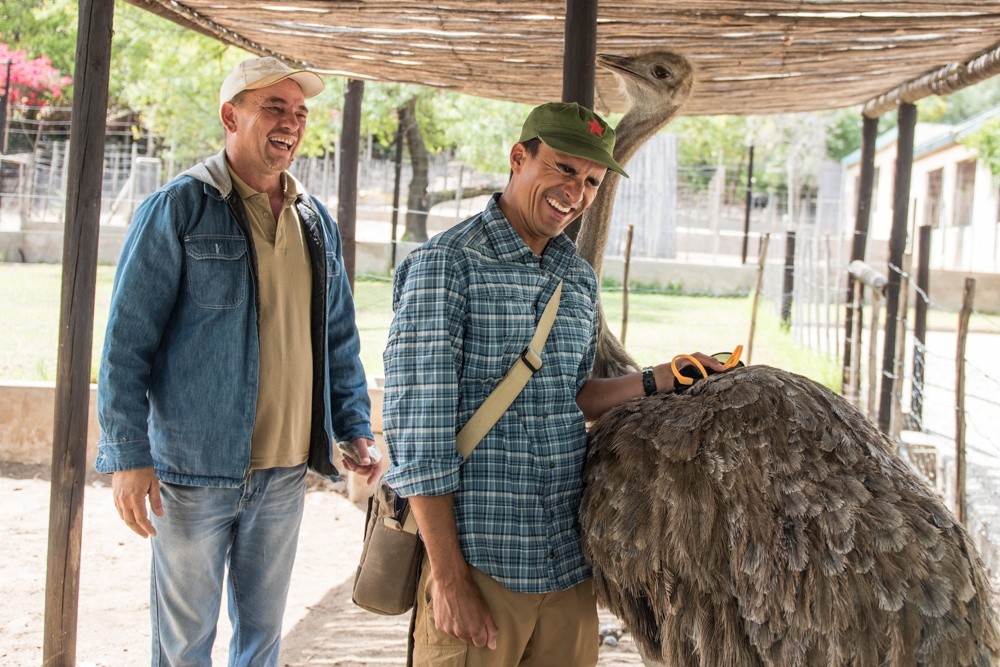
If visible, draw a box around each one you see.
[382,101,723,667]
[95,57,382,667]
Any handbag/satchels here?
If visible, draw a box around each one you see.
[351,472,431,615]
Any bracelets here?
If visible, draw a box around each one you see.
[641,366,658,398]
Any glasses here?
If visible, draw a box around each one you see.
[671,352,746,392]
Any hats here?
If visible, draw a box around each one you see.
[518,102,630,180]
[220,57,326,107]
